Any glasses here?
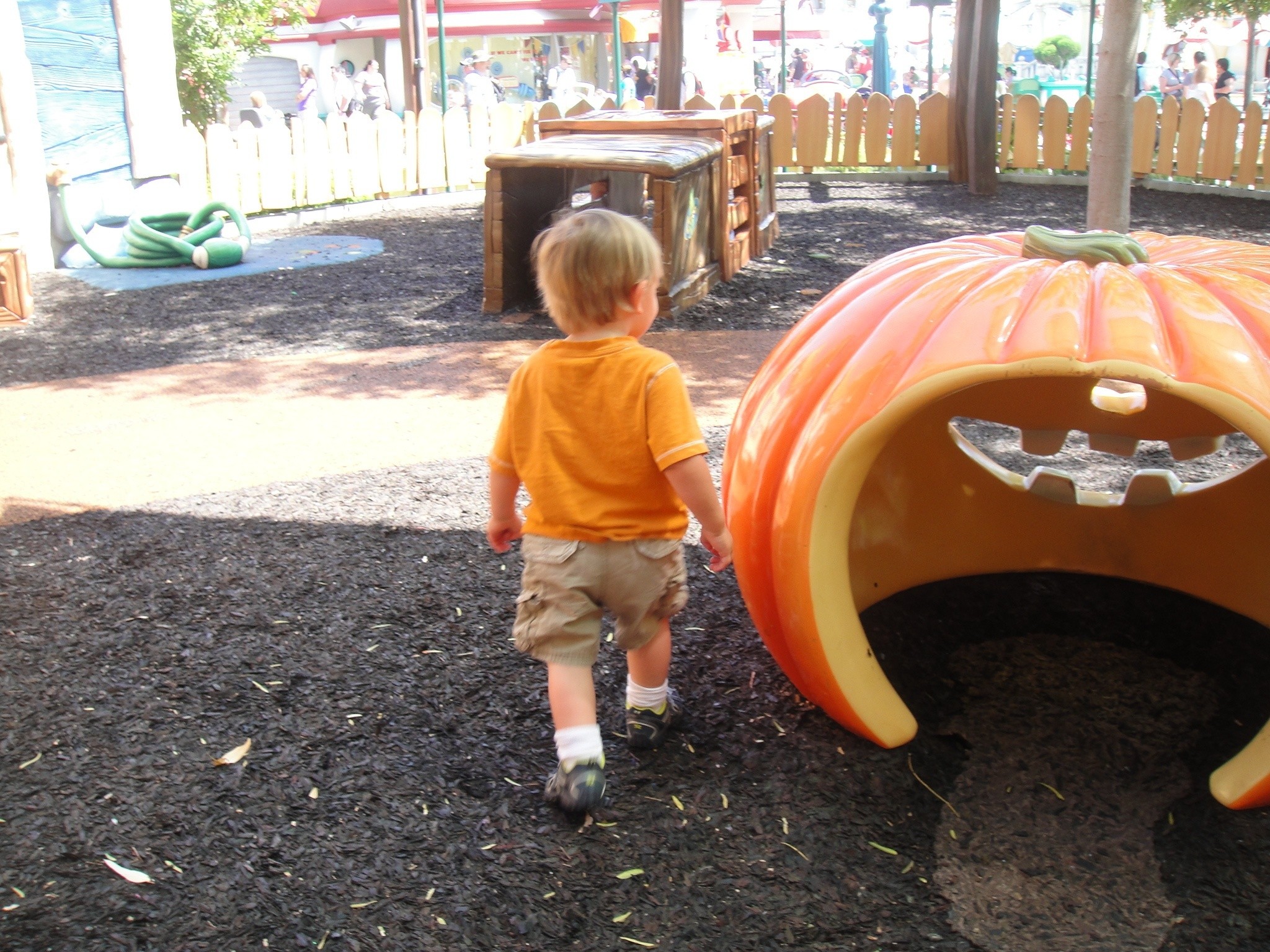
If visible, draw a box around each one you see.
[565,62,572,65]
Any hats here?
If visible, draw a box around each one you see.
[470,49,494,64]
[861,50,868,54]
[459,57,474,66]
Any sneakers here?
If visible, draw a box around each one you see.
[625,684,685,748]
[546,752,607,807]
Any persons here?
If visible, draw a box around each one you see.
[1159,53,1187,114]
[354,60,390,117]
[620,56,696,110]
[458,50,575,110]
[1135,51,1236,116]
[460,51,500,110]
[295,60,390,117]
[785,47,1016,112]
[485,207,734,814]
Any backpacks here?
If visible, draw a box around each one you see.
[1135,65,1145,96]
[682,71,705,97]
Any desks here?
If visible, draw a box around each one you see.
[482,110,779,322]
